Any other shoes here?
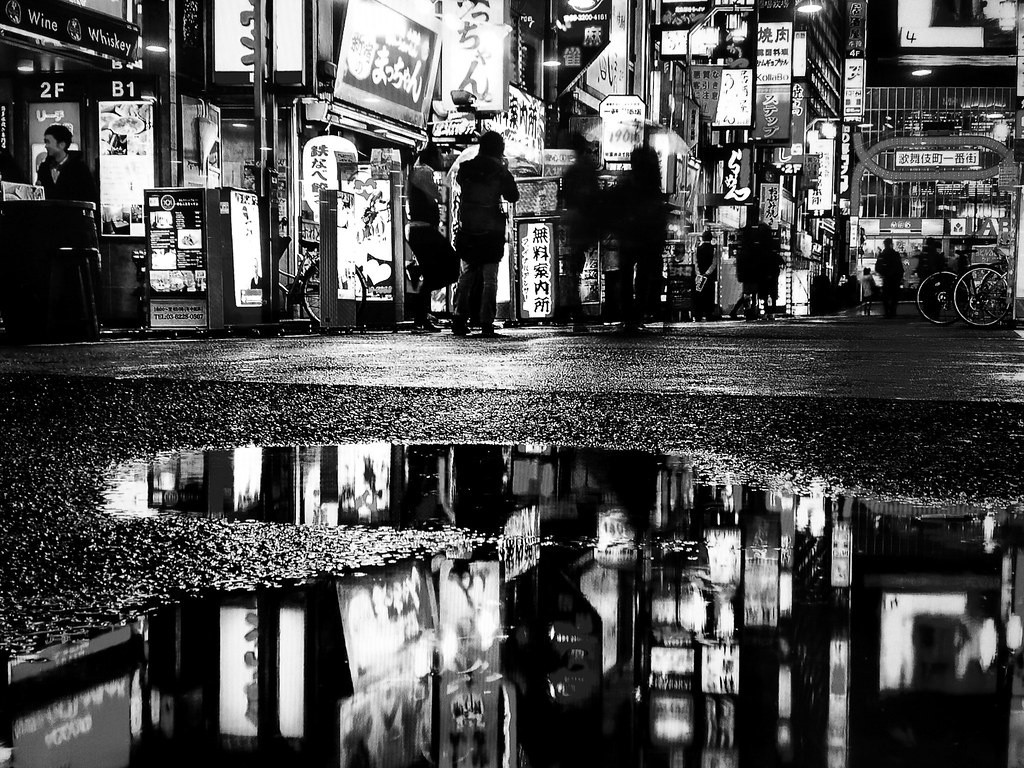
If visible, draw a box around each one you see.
[451,320,471,336]
[413,321,441,333]
[615,321,649,332]
[483,326,494,337]
[407,265,422,291]
[574,313,590,331]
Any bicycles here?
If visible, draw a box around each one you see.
[273,233,371,332]
[743,290,778,323]
[954,245,1014,329]
[917,249,1011,326]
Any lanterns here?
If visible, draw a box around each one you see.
[303,115,357,226]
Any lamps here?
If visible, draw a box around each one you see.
[724,12,741,31]
[451,90,477,111]
[797,0,822,12]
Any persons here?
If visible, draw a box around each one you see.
[814,269,832,315]
[452,130,520,337]
[917,238,942,322]
[602,145,670,335]
[860,267,879,317]
[875,238,905,319]
[692,231,722,322]
[729,226,759,321]
[35,125,98,201]
[674,242,689,264]
[553,141,606,333]
[406,144,460,334]
[753,223,785,321]
